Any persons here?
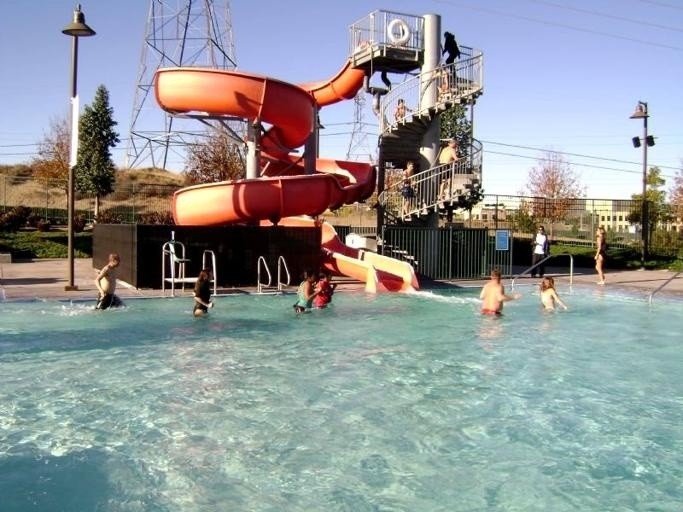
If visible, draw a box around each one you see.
[478,267,522,319]
[193,268,215,318]
[438,139,464,199]
[433,64,449,95]
[396,99,417,127]
[294,269,324,313]
[528,226,550,278]
[91,252,127,312]
[442,31,460,82]
[402,161,417,217]
[312,270,337,310]
[593,227,607,284]
[539,276,568,314]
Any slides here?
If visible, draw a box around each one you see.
[155,40,419,293]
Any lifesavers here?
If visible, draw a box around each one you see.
[386,18,411,46]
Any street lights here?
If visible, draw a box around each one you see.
[629,100,650,260]
[62,4,96,291]
